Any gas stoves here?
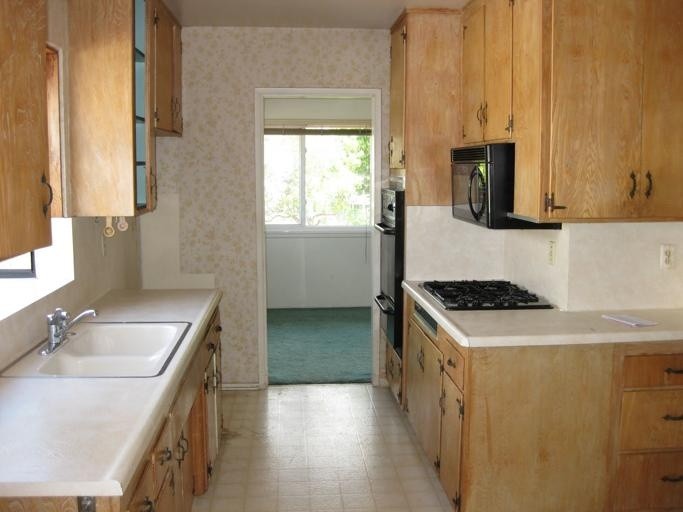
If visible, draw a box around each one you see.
[419,280,552,310]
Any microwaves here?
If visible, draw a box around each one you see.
[451,143,563,230]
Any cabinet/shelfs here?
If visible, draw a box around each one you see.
[388,1,683,226]
[0,308,223,511]
[0,0,51,261]
[64,1,184,220]
[399,292,683,512]
[383,341,401,404]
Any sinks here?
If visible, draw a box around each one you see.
[66,320,191,357]
[40,353,158,378]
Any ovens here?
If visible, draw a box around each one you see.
[374,188,405,359]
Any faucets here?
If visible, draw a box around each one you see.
[63,308,100,331]
